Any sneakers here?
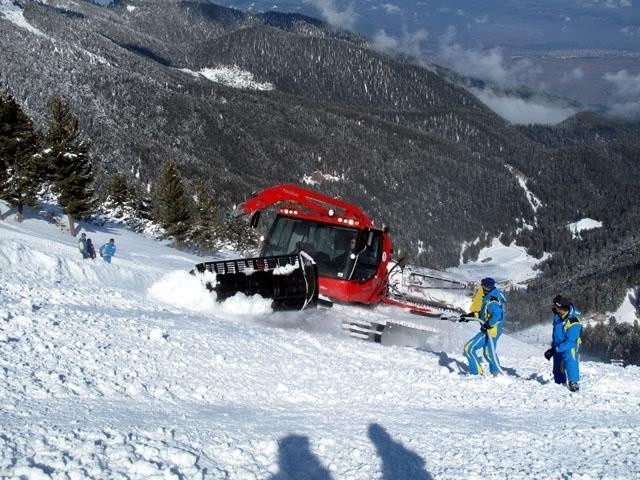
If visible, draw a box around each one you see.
[569,382,579,391]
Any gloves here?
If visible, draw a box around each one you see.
[459,312,474,323]
[544,348,554,361]
[480,324,489,335]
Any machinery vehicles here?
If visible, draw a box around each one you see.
[188,182,466,344]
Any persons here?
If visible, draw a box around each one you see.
[457,277,509,376]
[79,232,116,264]
[543,295,583,391]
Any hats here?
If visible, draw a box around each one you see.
[481,278,495,287]
[552,294,569,309]
